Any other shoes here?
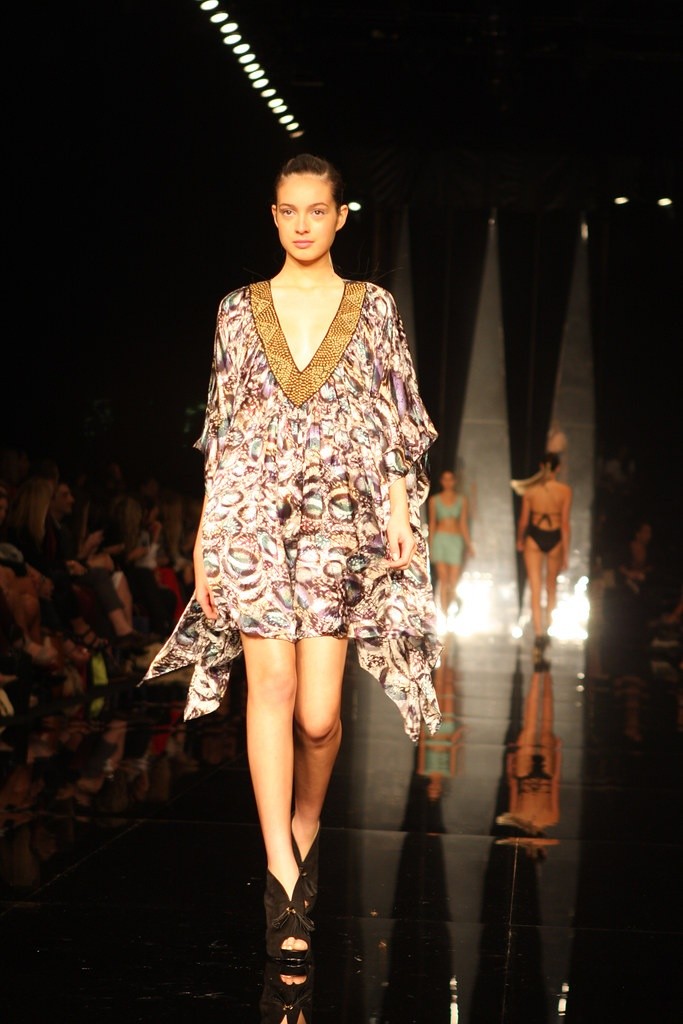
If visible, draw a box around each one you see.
[289,809,321,916]
[263,868,316,967]
[536,635,550,652]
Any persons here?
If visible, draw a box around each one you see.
[593,517,682,652]
[418,651,462,801]
[585,653,683,757]
[0,655,243,890]
[267,962,319,1024]
[518,455,572,645]
[135,154,438,961]
[503,649,562,861]
[429,473,473,608]
[1,439,202,718]
